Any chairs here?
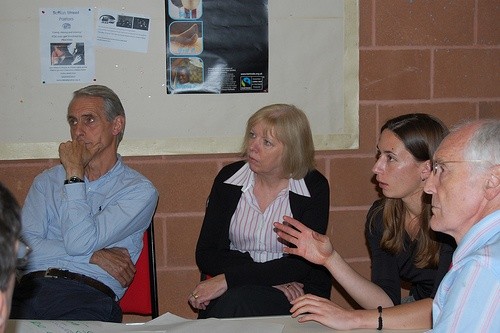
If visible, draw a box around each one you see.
[116,196,161,321]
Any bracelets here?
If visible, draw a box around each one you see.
[377,306,383,330]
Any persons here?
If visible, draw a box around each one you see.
[272,114,458,331]
[0,180,23,333]
[423,120,500,333]
[8,85,159,323]
[169,24,204,90]
[186,103,331,319]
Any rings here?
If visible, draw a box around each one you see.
[286,285,291,289]
[191,291,199,299]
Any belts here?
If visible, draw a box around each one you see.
[20,270,118,303]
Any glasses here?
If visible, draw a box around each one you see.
[0,220,32,269]
[431,157,487,176]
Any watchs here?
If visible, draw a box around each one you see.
[64,176,85,185]
